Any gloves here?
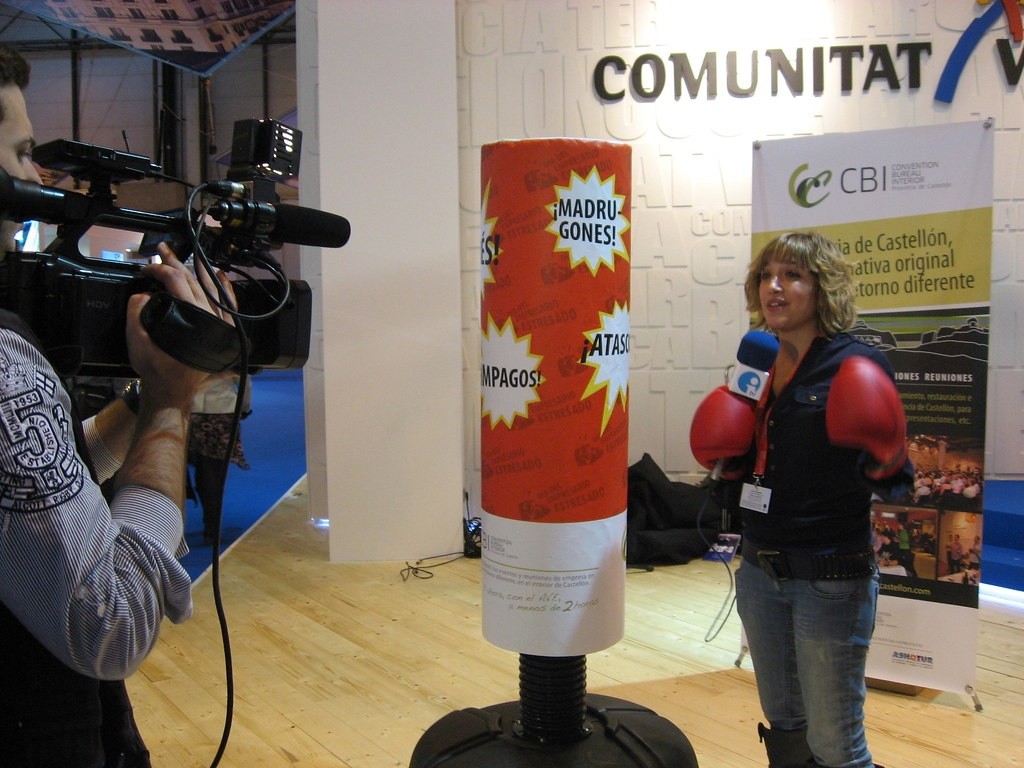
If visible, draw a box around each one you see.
[825,354,910,479]
[690,385,759,470]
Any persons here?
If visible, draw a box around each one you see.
[0,40,239,768]
[946,533,981,574]
[690,232,916,768]
[870,517,918,577]
[912,462,981,507]
[189,370,252,541]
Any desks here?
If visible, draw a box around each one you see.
[913,550,936,580]
[938,570,981,586]
[877,564,907,576]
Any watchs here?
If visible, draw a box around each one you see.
[121,378,141,416]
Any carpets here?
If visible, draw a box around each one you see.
[176,368,308,590]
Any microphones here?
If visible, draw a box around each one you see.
[712,329,780,481]
[212,200,351,247]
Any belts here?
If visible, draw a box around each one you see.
[738,535,877,581]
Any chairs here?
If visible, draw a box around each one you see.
[918,489,980,506]
